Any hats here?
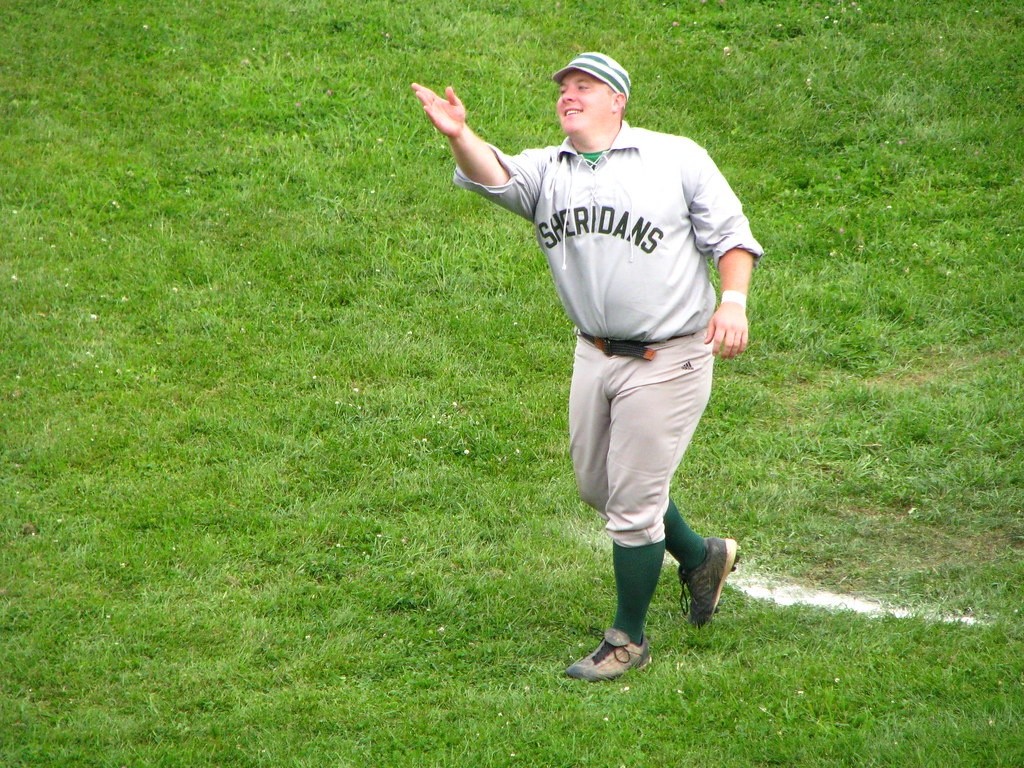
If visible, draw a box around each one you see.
[553,51,632,102]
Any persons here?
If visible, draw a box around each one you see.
[410,51,765,682]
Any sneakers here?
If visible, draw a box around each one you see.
[565,627,653,682]
[678,536,741,627]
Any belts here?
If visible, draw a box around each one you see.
[580,330,694,360]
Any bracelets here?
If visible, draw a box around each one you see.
[721,290,747,309]
